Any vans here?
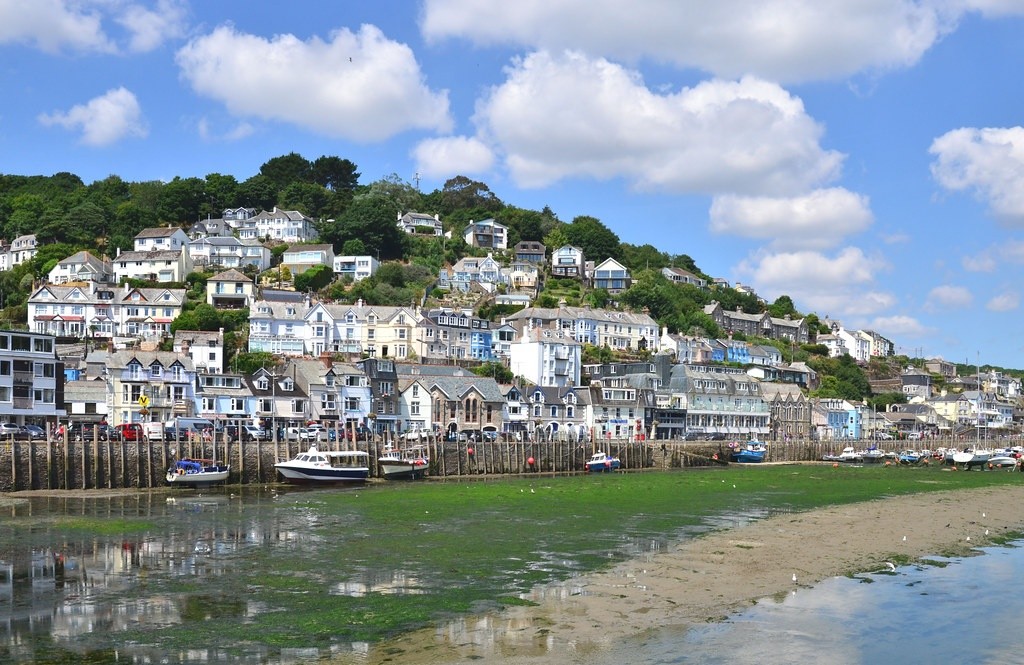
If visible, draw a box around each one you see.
[908,433,921,440]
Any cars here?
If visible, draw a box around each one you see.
[868,432,893,441]
[681,432,698,441]
[399,429,522,441]
[0,416,373,441]
[705,432,725,441]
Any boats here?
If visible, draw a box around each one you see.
[165,457,230,485]
[378,440,430,477]
[822,441,1024,466]
[586,450,620,472]
[274,442,369,483]
[732,440,766,463]
